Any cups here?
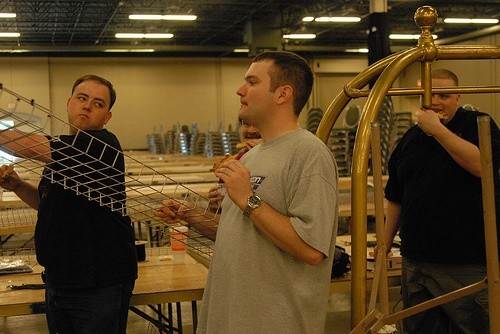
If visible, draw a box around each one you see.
[169,226,188,250]
[135,244,146,261]
[0,248,36,265]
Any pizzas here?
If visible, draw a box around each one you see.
[1,164,13,180]
[419,106,449,119]
[210,151,245,183]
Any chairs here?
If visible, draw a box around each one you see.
[146,104,417,177]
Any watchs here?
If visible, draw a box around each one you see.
[243,195,263,218]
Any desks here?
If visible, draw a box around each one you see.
[0,151,500,334]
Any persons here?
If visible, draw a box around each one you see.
[373,67,500,334]
[207,116,264,213]
[152,51,339,334]
[0,75,137,334]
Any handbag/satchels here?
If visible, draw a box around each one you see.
[331,245,349,278]
